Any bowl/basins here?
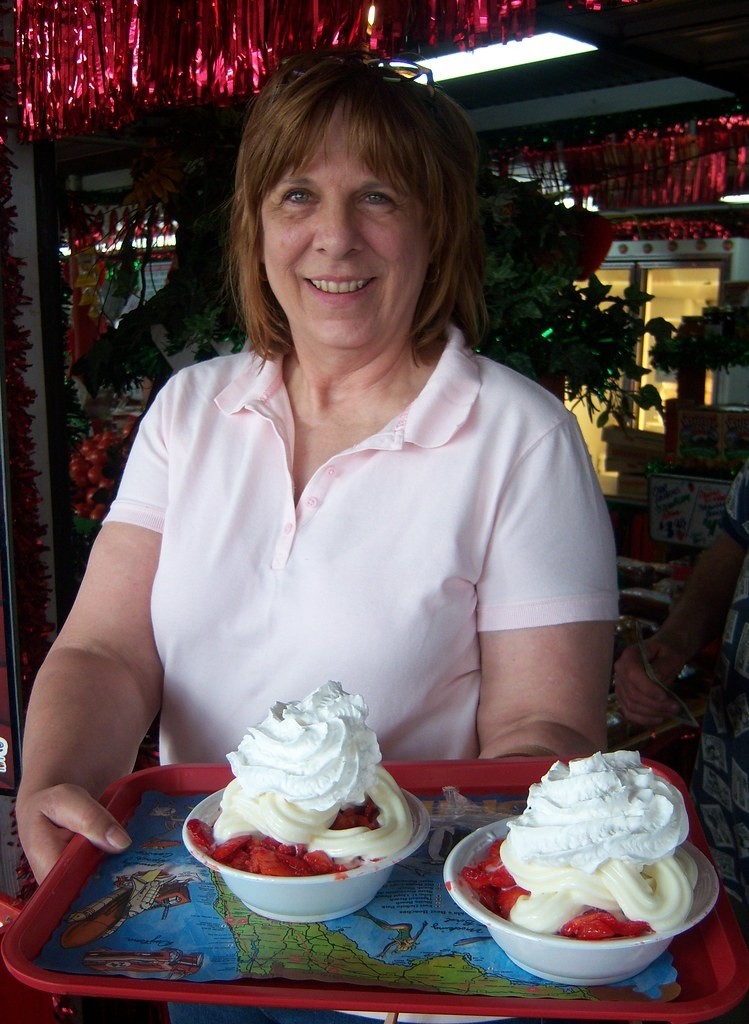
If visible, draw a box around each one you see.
[442,814,720,987]
[181,787,431,923]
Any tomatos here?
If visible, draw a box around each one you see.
[71,422,136,522]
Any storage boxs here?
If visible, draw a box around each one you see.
[601,398,749,547]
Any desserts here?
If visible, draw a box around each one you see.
[458,750,699,940]
[186,679,414,876]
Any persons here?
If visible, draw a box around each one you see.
[613,461,749,1024]
[14,66,617,1024]
